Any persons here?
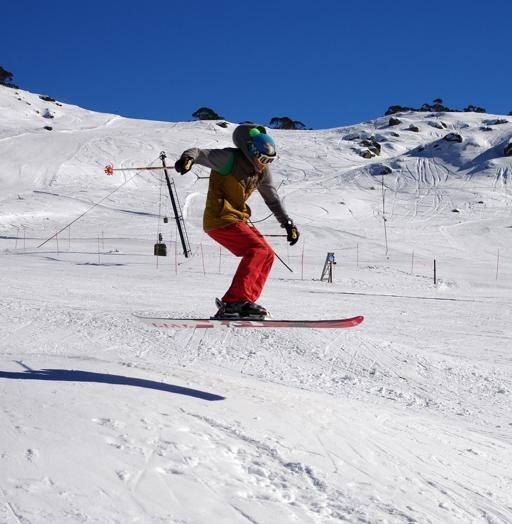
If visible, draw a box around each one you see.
[174,124,300,319]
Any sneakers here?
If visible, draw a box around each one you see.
[225,301,267,315]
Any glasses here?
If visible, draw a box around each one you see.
[249,138,276,165]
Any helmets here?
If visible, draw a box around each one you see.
[250,134,276,156]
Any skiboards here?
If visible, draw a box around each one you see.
[130,316,367,329]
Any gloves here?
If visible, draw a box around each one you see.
[175,157,194,175]
[285,221,299,245]
